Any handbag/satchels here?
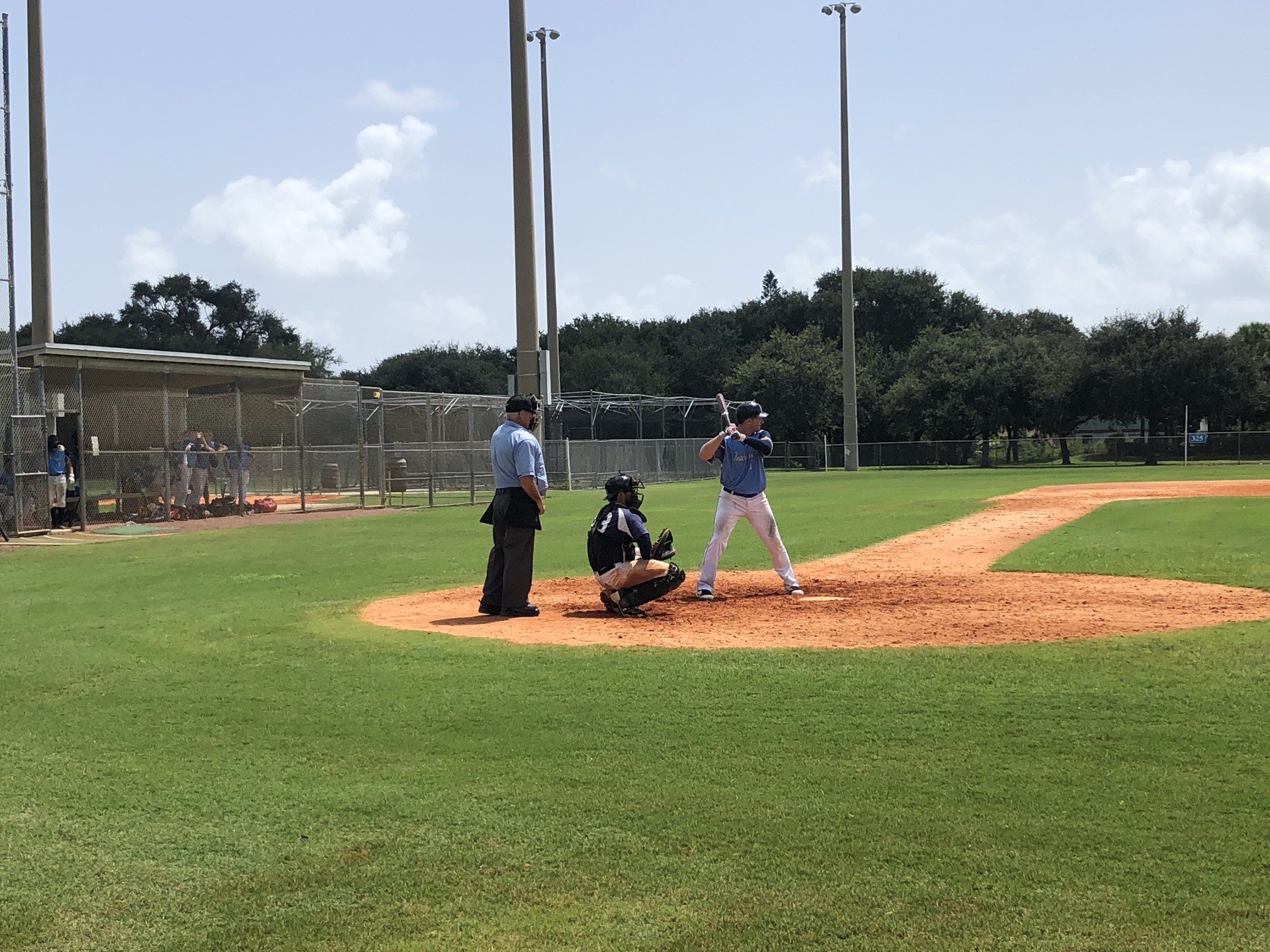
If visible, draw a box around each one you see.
[479,496,494,525]
[254,498,277,512]
[503,490,542,530]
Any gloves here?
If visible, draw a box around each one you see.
[68,474,75,484]
[719,423,736,440]
[726,427,746,442]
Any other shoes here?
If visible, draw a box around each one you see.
[478,596,501,614]
[500,602,540,616]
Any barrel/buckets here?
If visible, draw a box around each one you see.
[320,462,338,488]
[385,457,408,492]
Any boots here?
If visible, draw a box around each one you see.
[58,506,67,527]
[51,506,55,528]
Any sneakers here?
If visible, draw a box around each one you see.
[787,586,804,595]
[600,589,621,613]
[610,590,646,618]
[697,589,714,601]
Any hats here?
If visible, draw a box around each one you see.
[209,453,218,468]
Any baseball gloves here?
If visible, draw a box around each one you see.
[651,528,675,560]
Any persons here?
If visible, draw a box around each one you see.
[0,434,75,530]
[697,402,803,600]
[478,393,549,616]
[586,474,685,618]
[134,430,260,506]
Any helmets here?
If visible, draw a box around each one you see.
[735,400,769,424]
[506,393,540,414]
[48,434,61,448]
[602,474,638,500]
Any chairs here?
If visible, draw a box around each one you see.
[119,476,146,516]
[0,485,82,534]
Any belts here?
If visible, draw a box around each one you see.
[496,487,525,495]
[723,487,762,498]
[50,473,66,476]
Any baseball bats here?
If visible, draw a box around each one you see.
[716,393,737,440]
